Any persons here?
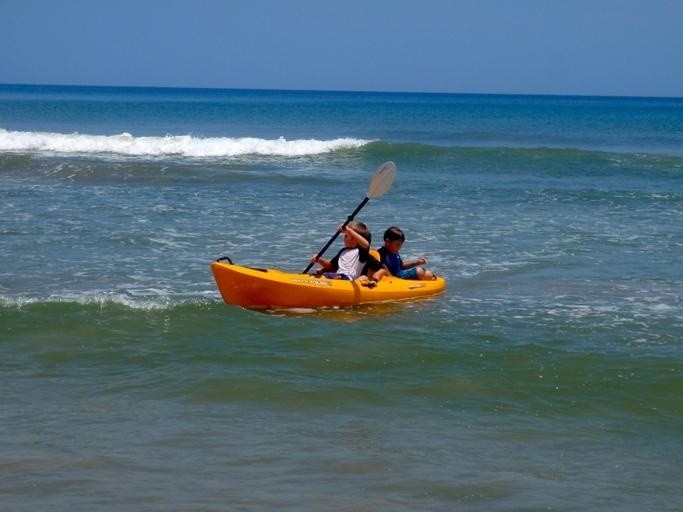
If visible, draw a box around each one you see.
[309,220,373,281]
[376,225,434,281]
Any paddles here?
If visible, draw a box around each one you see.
[302,161,396,274]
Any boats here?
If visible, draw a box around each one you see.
[208,253,450,316]
